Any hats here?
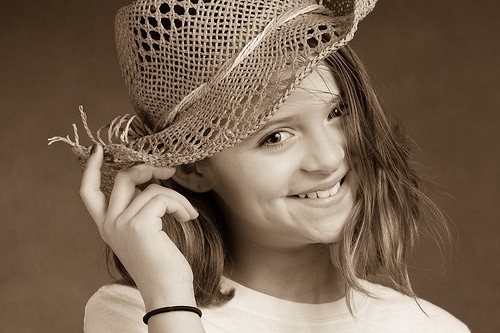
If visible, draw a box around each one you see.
[48,0,380,206]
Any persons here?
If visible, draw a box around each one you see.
[45,0,471,332]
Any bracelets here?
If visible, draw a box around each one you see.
[142,305,202,326]
